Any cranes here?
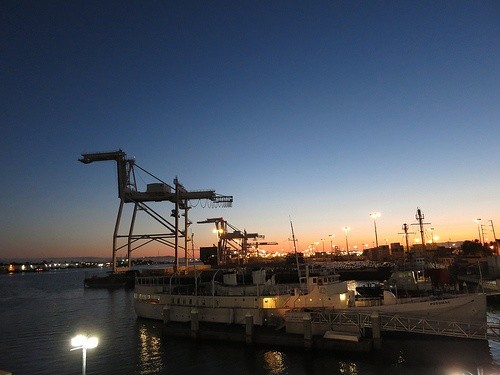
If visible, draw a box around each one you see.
[227,238,278,265]
[195,217,265,265]
[77,148,234,271]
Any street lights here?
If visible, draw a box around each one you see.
[343,226,350,255]
[321,238,326,252]
[328,234,334,253]
[368,213,381,248]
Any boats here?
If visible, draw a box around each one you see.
[131,221,500,340]
